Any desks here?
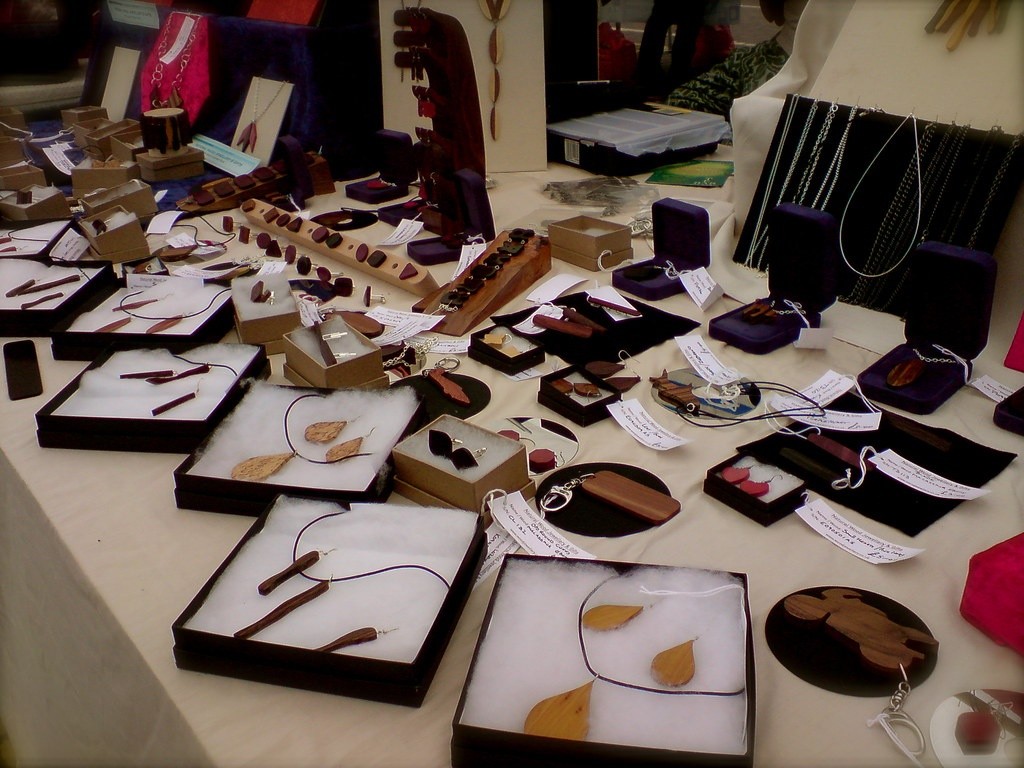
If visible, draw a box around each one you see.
[0,145,1024,768]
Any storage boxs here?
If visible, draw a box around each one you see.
[0,261,117,337]
[83,118,140,153]
[80,180,159,216]
[135,146,204,181]
[51,279,235,361]
[73,117,114,147]
[83,149,111,162]
[60,106,109,130]
[171,495,489,708]
[230,102,998,527]
[70,163,141,189]
[173,383,430,517]
[78,204,150,263]
[34,341,272,454]
[0,164,47,190]
[0,184,72,220]
[0,216,81,262]
[450,551,756,768]
[110,130,147,162]
[0,106,30,138]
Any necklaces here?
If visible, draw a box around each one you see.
[229,393,372,482]
[231,510,450,642]
[743,92,1024,307]
[743,300,807,327]
[522,573,744,744]
[885,346,966,388]
[150,11,202,109]
[237,77,286,152]
